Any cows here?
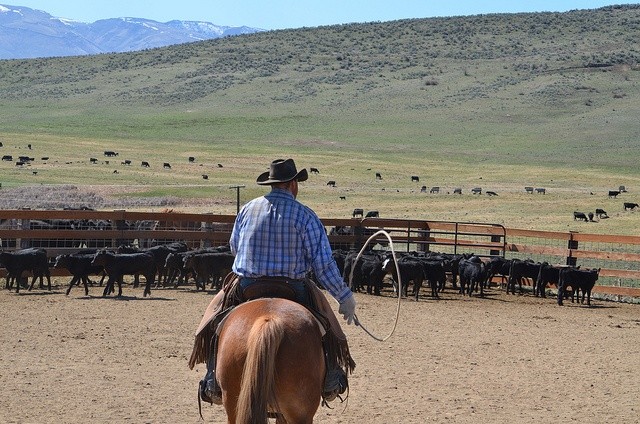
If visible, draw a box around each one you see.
[588,211,594,222]
[340,196,345,200]
[164,163,171,169]
[604,214,609,218]
[535,188,546,195]
[574,211,588,222]
[90,157,98,162]
[608,190,621,199]
[15,162,25,167]
[596,208,607,219]
[18,156,29,161]
[2,156,12,161]
[121,162,126,165]
[525,186,533,193]
[141,161,150,168]
[0,242,236,299]
[202,174,208,179]
[411,175,419,182]
[376,173,381,179]
[472,188,481,194]
[29,158,34,161]
[28,144,31,149]
[619,185,626,192]
[310,168,319,174]
[486,191,498,196]
[105,160,110,164]
[105,151,119,158]
[218,164,223,167]
[0,207,162,242]
[454,188,461,194]
[624,202,639,210]
[430,186,439,193]
[329,249,602,306]
[114,169,117,173]
[327,181,335,187]
[215,297,326,424]
[41,157,49,161]
[421,185,426,193]
[33,172,38,175]
[189,157,195,163]
[125,160,131,165]
[365,210,379,218]
[353,208,363,217]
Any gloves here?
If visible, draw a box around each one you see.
[338,296,357,325]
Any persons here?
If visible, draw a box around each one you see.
[204,158,357,406]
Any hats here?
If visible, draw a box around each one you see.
[255,158,309,186]
